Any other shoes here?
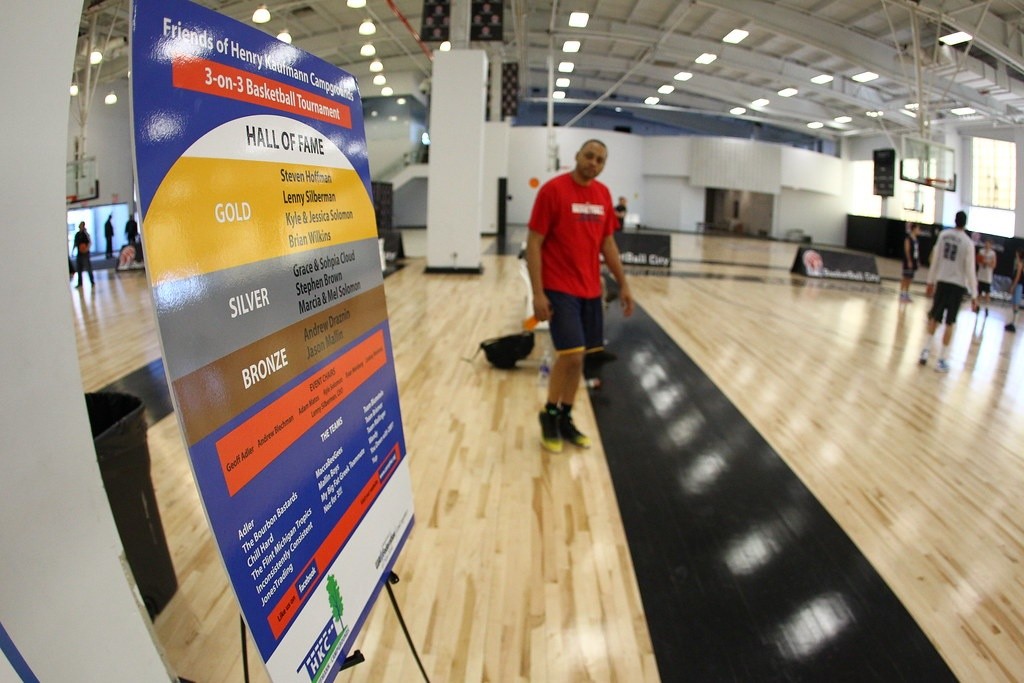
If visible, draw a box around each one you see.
[558,413,588,448]
[920,350,929,364]
[935,359,950,373]
[539,409,563,453]
[1005,324,1016,333]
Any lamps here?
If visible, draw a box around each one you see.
[369,56,384,73]
[359,16,377,36]
[252,2,272,23]
[360,40,377,57]
[276,27,292,44]
[104,90,117,105]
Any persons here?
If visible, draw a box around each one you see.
[1005,248,1024,332]
[125,215,139,243]
[899,223,920,302]
[527,139,635,453]
[105,214,114,259]
[71,221,95,289]
[975,239,996,316]
[919,211,979,374]
[612,197,626,228]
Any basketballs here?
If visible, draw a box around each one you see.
[79,243,86,252]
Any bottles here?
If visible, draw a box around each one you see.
[524,305,555,330]
[538,350,552,385]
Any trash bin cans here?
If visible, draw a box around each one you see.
[84,392,179,621]
